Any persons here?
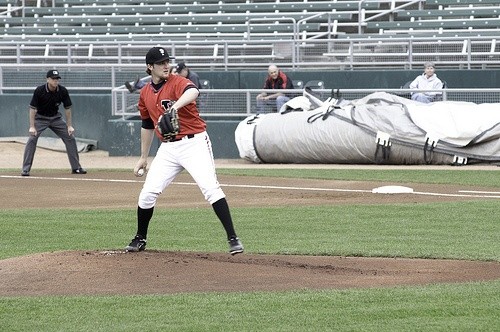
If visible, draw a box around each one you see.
[21,70,87,175]
[410,63,443,103]
[126,47,244,255]
[124,62,201,111]
[257,65,295,113]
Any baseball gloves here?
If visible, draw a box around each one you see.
[156,108,180,141]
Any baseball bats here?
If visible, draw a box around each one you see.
[137,168,144,177]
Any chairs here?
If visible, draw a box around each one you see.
[199,80,210,89]
[0,0,500,69]
[400,81,447,100]
[291,80,324,100]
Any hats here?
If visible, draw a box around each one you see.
[174,61,185,73]
[47,70,61,79]
[146,47,175,63]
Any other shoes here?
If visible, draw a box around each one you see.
[72,168,87,174]
[125,81,136,93]
[21,170,29,176]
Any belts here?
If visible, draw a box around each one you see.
[162,133,194,142]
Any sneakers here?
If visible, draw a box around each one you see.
[125,234,147,251]
[228,236,244,256]
